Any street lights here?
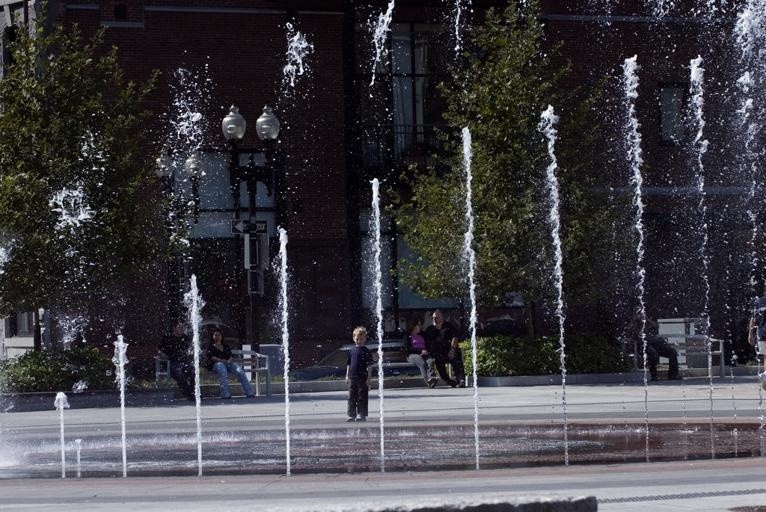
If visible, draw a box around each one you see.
[222,103,280,358]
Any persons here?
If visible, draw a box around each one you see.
[748,290,766,376]
[399,317,441,387]
[422,309,466,388]
[341,325,375,422]
[204,327,255,401]
[165,321,203,401]
[622,303,682,381]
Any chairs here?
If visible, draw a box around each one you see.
[340,341,469,391]
[153,348,271,401]
[617,317,726,385]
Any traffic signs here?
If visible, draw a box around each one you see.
[231,218,268,235]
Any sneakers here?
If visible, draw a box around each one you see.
[188,391,256,400]
[427,377,466,389]
[347,414,366,422]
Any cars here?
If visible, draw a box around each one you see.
[180,320,241,349]
[290,339,403,380]
